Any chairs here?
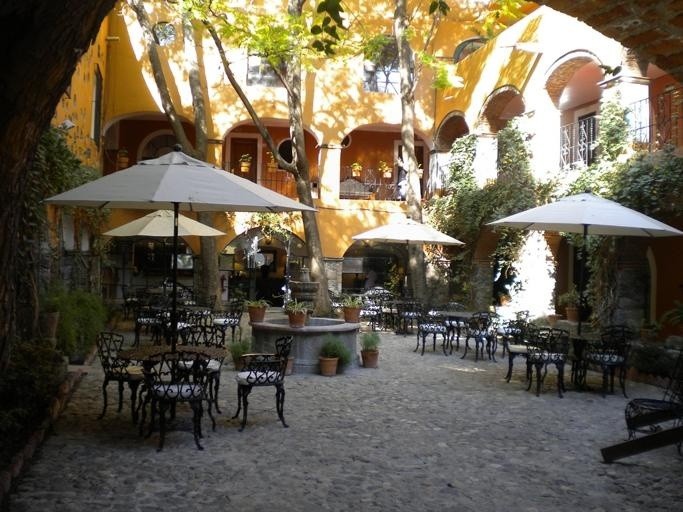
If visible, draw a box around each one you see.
[361,291,630,402]
[175,341,228,414]
[140,351,210,452]
[625,348,683,441]
[232,336,293,432]
[121,280,241,348]
[96,333,147,426]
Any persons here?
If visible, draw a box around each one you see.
[365,265,379,288]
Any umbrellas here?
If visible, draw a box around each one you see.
[484,192,683,334]
[101,210,227,296]
[37,144,319,366]
[351,216,467,261]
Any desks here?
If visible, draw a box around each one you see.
[120,345,229,432]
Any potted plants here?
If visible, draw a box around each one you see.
[238,154,252,173]
[340,293,365,323]
[318,333,352,376]
[639,317,661,345]
[265,151,278,174]
[283,297,313,328]
[243,298,269,322]
[278,345,295,376]
[226,328,256,371]
[358,330,380,368]
[559,283,584,322]
[350,161,363,177]
[378,159,392,178]
